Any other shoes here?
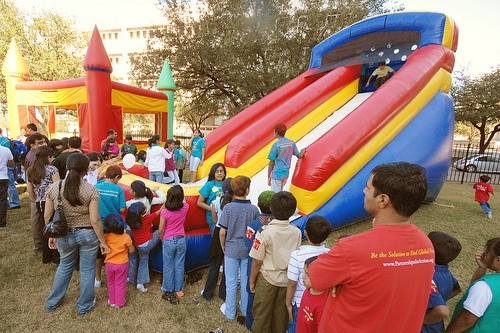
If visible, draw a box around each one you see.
[11,206,20,209]
[220,303,227,315]
[488,211,493,220]
[201,289,209,303]
[237,316,245,325]
[94,278,102,288]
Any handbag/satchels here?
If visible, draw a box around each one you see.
[43,181,67,237]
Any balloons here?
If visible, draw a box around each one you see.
[123,154,135,170]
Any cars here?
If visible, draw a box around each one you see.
[453,153,500,174]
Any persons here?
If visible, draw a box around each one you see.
[187,130,205,185]
[285,215,351,333]
[197,162,226,237]
[92,164,166,308]
[310,161,435,333]
[365,60,396,90]
[219,175,260,320]
[473,175,495,220]
[445,237,500,333]
[145,135,187,184]
[268,123,306,194]
[100,129,120,160]
[28,136,111,315]
[237,190,276,331]
[248,191,302,333]
[120,134,147,163]
[420,231,462,333]
[0,123,50,227]
[158,185,189,297]
[201,178,235,303]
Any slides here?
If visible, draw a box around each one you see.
[195,66,434,215]
